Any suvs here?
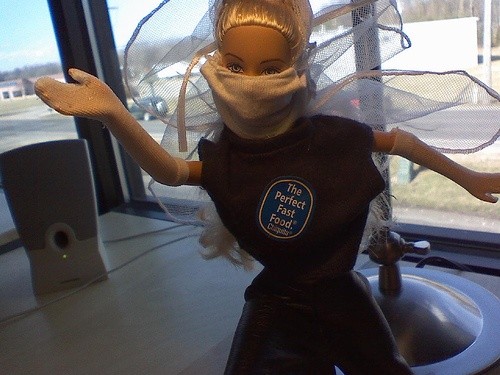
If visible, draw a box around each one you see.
[128,97,168,121]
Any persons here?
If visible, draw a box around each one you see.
[33,1,500,375]
